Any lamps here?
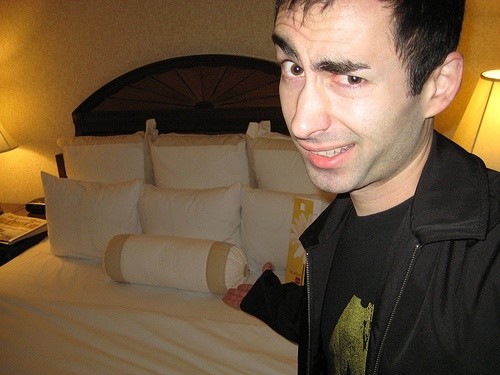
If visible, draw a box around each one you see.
[0,122,20,214]
[450,69,500,174]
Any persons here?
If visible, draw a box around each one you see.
[223,1,500,375]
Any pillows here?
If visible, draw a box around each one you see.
[57,119,156,186]
[238,187,331,274]
[249,119,332,196]
[40,171,139,259]
[152,132,250,192]
[103,233,248,294]
[0,55,338,375]
[137,175,242,247]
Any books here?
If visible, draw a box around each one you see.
[0,213,48,245]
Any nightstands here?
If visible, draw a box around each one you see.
[0,203,46,265]
